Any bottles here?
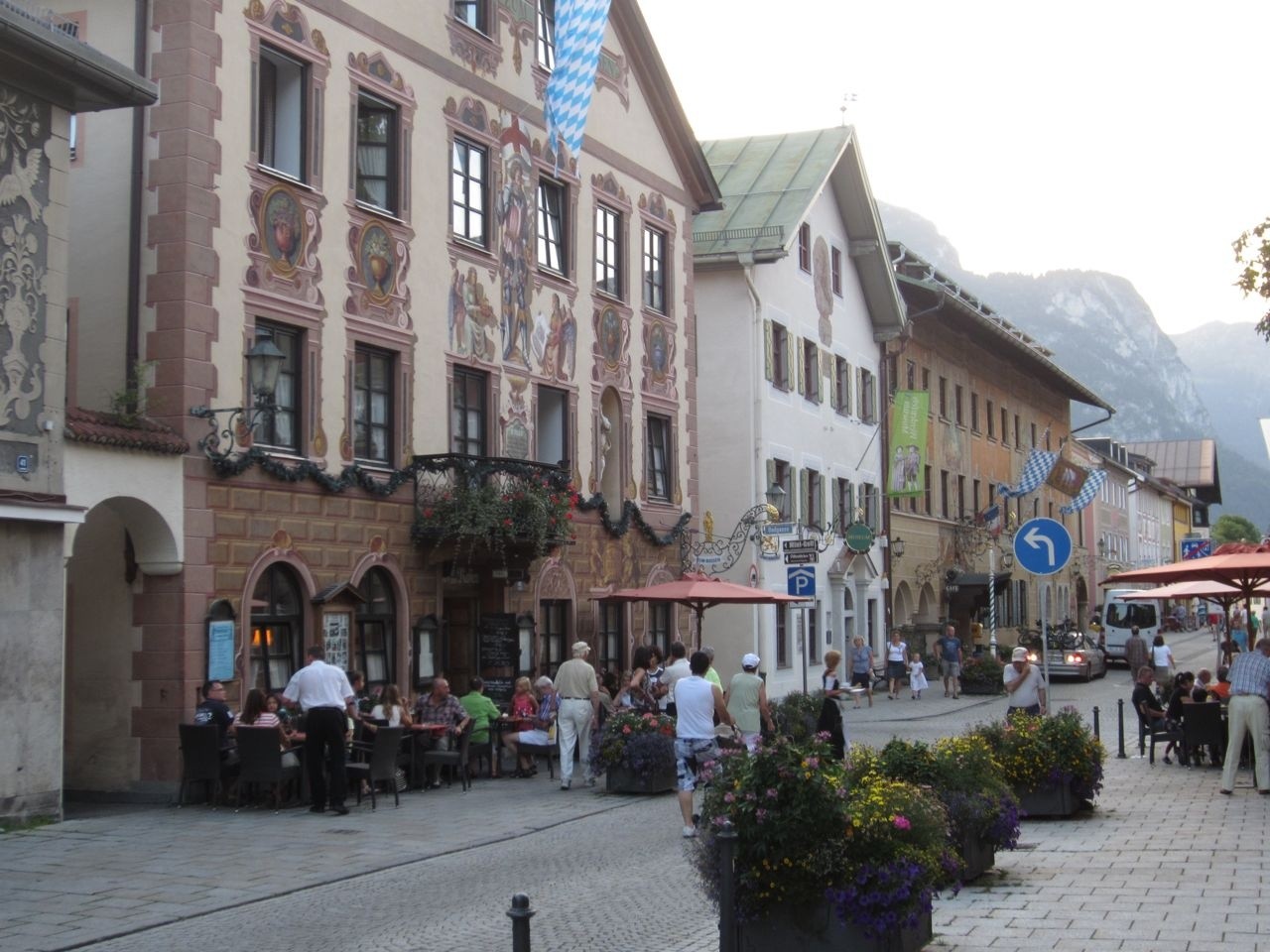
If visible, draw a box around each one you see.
[1206,695,1212,702]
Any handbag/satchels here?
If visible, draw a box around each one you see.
[1148,645,1155,668]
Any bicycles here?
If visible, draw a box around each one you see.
[1015,615,1084,649]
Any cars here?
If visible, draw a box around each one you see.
[1020,633,1108,683]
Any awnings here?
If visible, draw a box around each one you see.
[945,571,1014,598]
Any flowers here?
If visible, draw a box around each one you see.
[761,686,830,741]
[958,650,1004,687]
[581,707,677,788]
[412,469,581,581]
[680,703,1109,948]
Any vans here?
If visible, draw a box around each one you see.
[1094,588,1164,663]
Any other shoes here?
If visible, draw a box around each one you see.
[945,692,949,697]
[682,825,700,837]
[560,779,572,790]
[1219,789,1231,794]
[888,694,894,700]
[918,695,921,699]
[585,779,596,786]
[329,804,348,814]
[953,694,959,699]
[912,695,915,699]
[510,769,532,778]
[526,765,538,775]
[1259,790,1270,794]
[433,779,443,788]
[363,786,380,797]
[309,806,325,813]
[854,705,860,709]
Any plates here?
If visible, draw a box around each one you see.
[840,687,852,690]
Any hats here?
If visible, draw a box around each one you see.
[572,641,591,651]
[1012,647,1029,663]
[742,654,761,669]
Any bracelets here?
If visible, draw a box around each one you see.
[1041,705,1046,706]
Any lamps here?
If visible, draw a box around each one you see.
[749,481,787,542]
[887,537,908,562]
[999,553,1014,572]
[1068,560,1082,581]
[188,328,290,464]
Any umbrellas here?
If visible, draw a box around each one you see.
[1098,537,1270,775]
[1113,535,1270,665]
[588,571,813,652]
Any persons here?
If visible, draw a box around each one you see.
[673,652,736,838]
[1220,639,1270,794]
[1125,625,1247,771]
[822,650,852,763]
[892,445,921,492]
[372,683,412,790]
[195,640,776,794]
[1003,647,1047,726]
[848,622,963,701]
[282,645,355,815]
[194,679,238,800]
[1209,602,1270,665]
[1173,598,1191,632]
[1198,598,1207,626]
[233,689,301,804]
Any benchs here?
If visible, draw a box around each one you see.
[871,657,911,695]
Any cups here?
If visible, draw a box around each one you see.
[422,722,429,729]
[842,683,849,688]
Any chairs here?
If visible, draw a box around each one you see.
[176,701,560,814]
[1131,693,1227,771]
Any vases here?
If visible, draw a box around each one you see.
[1018,772,1083,819]
[605,763,678,796]
[955,835,995,882]
[960,680,1001,695]
[733,891,933,952]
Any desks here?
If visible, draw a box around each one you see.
[1218,699,1254,771]
[404,722,450,790]
[491,715,533,780]
